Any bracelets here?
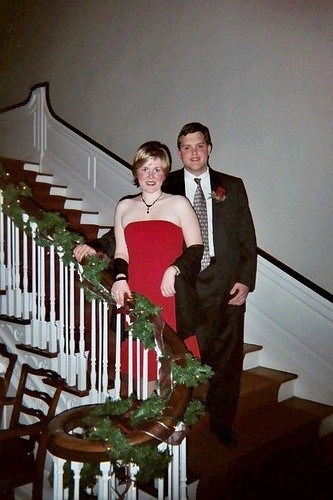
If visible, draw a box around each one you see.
[172,265,179,277]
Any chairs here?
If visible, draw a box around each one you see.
[0,344,66,500]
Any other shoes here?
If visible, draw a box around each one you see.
[209,425,237,447]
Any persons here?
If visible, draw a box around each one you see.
[73,123,257,448]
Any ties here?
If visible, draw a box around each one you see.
[193,178,210,272]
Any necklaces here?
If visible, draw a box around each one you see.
[140,192,163,214]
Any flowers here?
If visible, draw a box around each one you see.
[207,187,226,205]
